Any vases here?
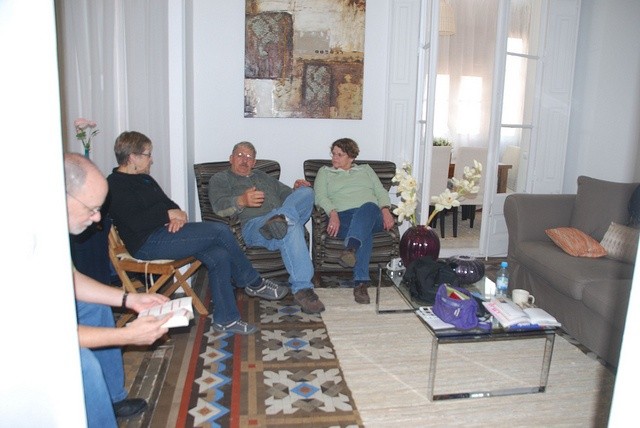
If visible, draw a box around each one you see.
[400,225,440,268]
[84,146,89,159]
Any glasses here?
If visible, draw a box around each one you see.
[137,151,152,158]
[233,152,254,161]
[67,191,102,217]
[329,151,348,159]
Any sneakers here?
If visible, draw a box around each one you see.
[294,287,325,314]
[213,319,259,336]
[258,214,288,241]
[244,277,289,300]
[353,283,370,305]
[338,249,356,269]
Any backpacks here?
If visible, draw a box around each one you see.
[400,257,460,304]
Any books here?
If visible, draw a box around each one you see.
[137,296,194,328]
[417,306,455,329]
[481,296,562,332]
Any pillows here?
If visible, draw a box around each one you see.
[600,222,640,264]
[545,227,607,257]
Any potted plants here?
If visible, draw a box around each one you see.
[432,137,454,164]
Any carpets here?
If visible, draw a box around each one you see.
[315,286,614,428]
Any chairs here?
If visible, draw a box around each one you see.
[504,145,520,192]
[451,146,488,237]
[108,224,210,328]
[428,146,451,239]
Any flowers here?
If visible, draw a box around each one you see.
[73,118,101,147]
[391,160,482,226]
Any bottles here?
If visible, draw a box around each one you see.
[390,243,402,278]
[496,261,510,300]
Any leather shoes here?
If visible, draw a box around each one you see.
[113,397,148,420]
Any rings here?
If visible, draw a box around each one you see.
[331,225,333,228]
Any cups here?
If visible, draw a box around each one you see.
[512,289,535,307]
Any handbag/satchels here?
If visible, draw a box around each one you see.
[432,282,480,330]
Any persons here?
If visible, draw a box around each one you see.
[61,152,175,428]
[208,141,325,314]
[313,138,395,304]
[99,131,288,336]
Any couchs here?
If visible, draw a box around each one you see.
[193,159,309,281]
[503,175,640,367]
[304,159,396,287]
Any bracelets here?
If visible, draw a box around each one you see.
[122,290,129,308]
